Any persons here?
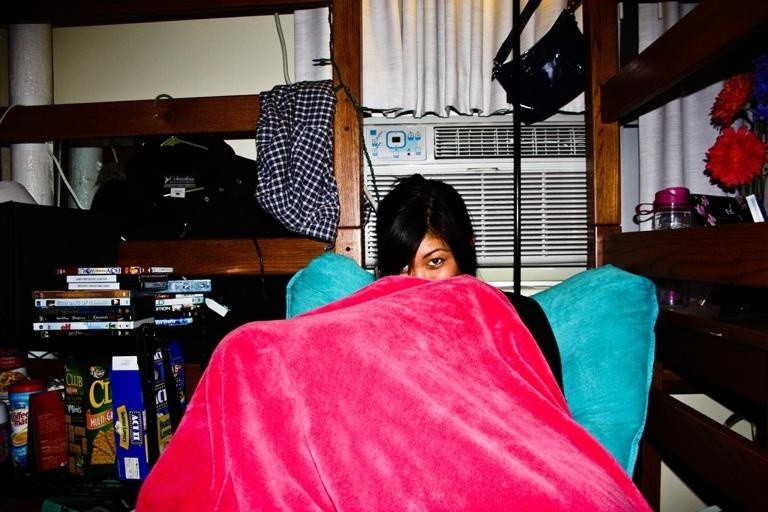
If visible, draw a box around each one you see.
[373,173,563,405]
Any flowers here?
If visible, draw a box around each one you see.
[705,75,767,201]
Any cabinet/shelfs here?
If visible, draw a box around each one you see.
[0,0,363,511]
[584,0,766,511]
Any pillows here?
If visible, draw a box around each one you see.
[286,250,660,485]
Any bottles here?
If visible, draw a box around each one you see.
[635,183,693,309]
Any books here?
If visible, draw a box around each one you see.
[32,260,213,342]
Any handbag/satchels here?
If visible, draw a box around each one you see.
[491,0,588,127]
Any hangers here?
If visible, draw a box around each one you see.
[152,94,206,151]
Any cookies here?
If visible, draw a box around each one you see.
[90,428,116,464]
